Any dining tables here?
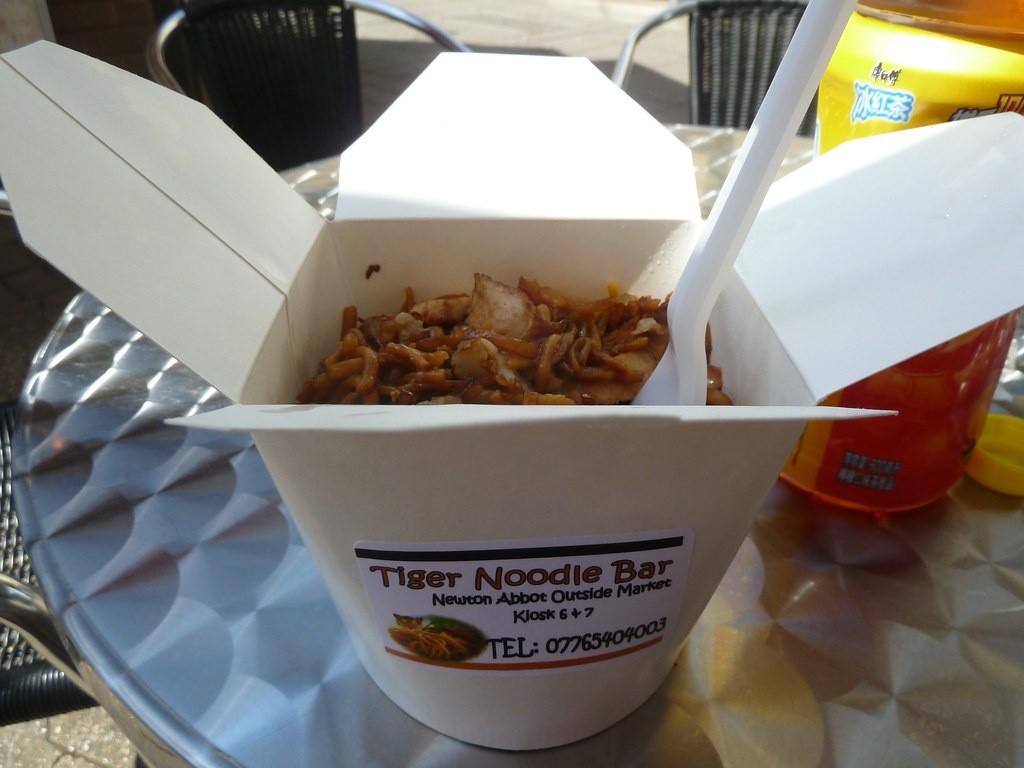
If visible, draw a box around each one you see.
[11,123,1024,767]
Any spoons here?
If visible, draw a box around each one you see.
[631,0,858,408]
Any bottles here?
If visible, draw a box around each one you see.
[779,0,1023,516]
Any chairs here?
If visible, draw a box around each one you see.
[608,1,820,138]
[144,1,476,174]
[0,397,104,731]
